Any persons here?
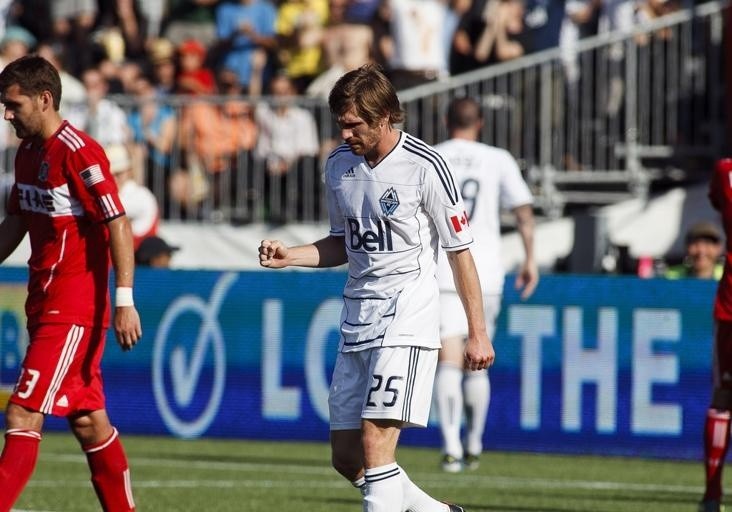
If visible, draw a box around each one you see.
[259,68,497,512]
[425,96,539,471]
[699,155,732,512]
[1,0,732,224]
[107,143,159,265]
[1,57,145,512]
[136,237,181,269]
[667,222,726,280]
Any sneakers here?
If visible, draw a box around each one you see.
[463,453,482,473]
[442,455,464,474]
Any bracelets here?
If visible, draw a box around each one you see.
[113,285,134,307]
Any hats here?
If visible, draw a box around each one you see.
[136,238,179,264]
[685,221,723,243]
